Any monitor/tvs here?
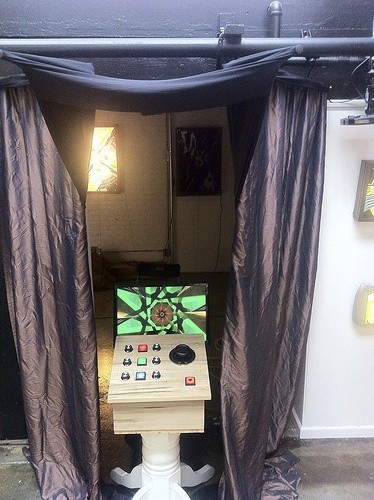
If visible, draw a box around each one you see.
[113,282,209,348]
